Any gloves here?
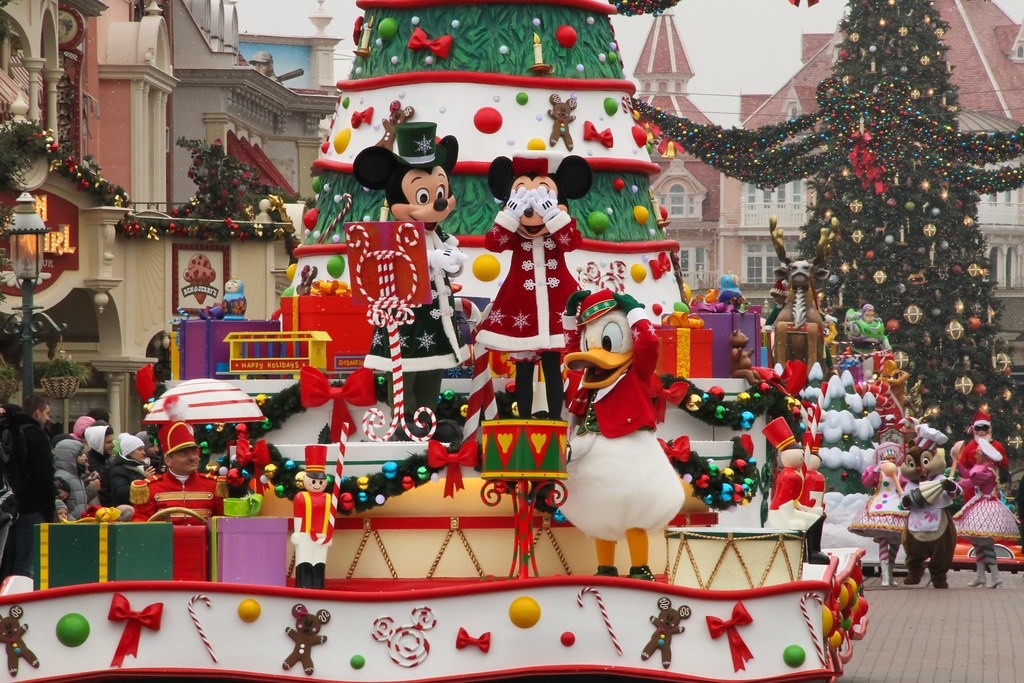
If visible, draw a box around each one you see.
[874,461,884,473]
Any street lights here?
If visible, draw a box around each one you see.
[2,190,52,411]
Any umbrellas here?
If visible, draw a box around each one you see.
[144,378,266,423]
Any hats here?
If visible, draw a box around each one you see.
[973,406,991,425]
[55,499,67,509]
[118,432,145,457]
[770,280,787,297]
[135,431,150,450]
[158,421,199,457]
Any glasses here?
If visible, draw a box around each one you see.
[974,425,990,431]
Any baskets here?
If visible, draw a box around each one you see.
[41,343,80,400]
[0,354,17,402]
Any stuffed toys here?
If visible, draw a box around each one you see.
[902,441,956,588]
[552,289,685,579]
[474,156,593,419]
[353,122,471,441]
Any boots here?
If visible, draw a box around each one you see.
[967,561,987,587]
[881,560,899,587]
[987,565,1003,588]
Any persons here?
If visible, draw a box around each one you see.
[0,395,164,598]
[133,424,222,525]
[847,303,893,357]
[958,411,1008,504]
[848,441,908,588]
[951,449,1019,589]
